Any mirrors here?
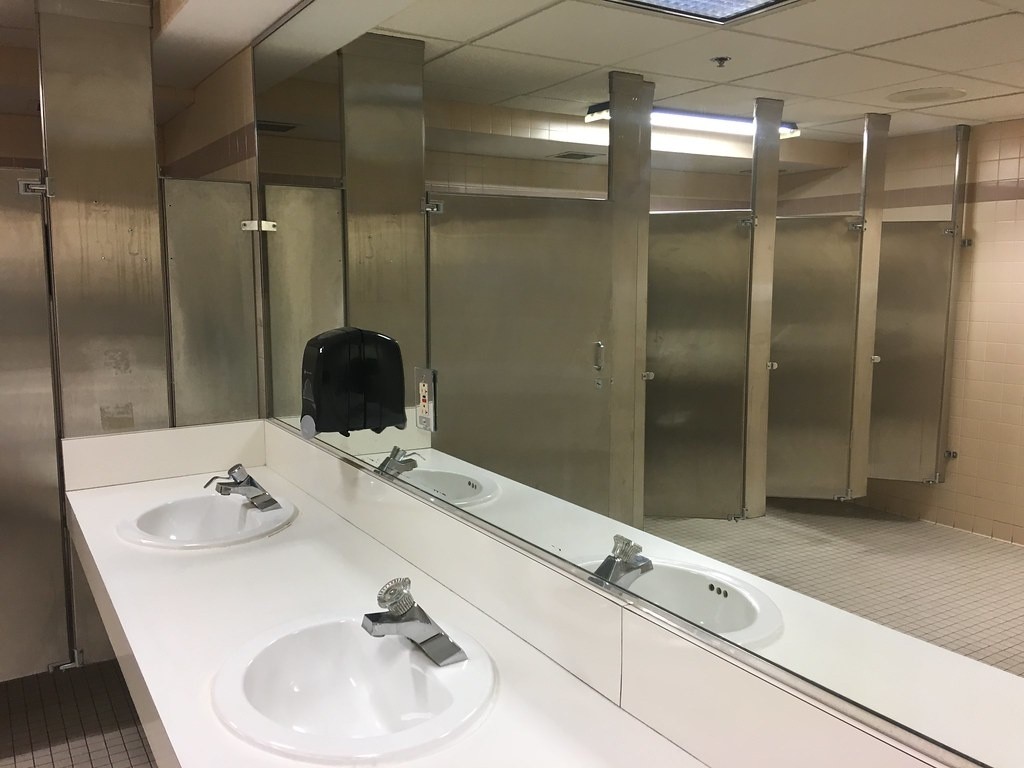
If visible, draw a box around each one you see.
[253,1,1023,768]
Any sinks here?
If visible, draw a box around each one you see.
[558,548,787,661]
[212,608,499,766]
[384,461,505,515]
[116,484,300,552]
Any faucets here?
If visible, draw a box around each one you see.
[589,534,655,595]
[359,575,472,669]
[203,461,284,512]
[377,444,429,476]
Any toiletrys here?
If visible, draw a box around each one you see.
[361,327,410,437]
[298,324,367,442]
